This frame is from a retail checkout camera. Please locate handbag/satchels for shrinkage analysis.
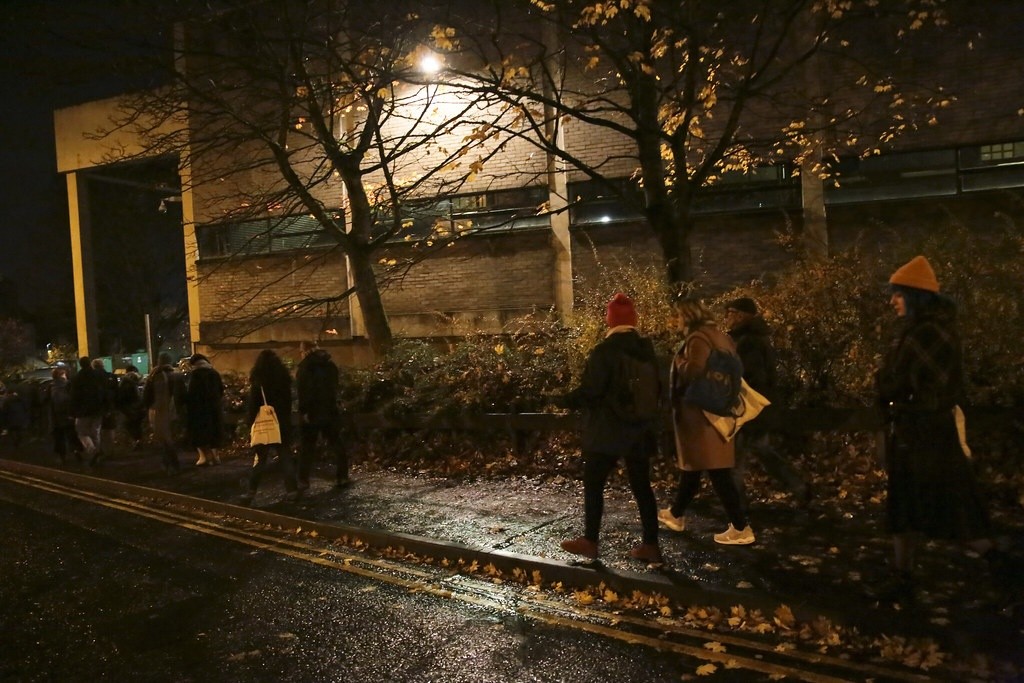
[702,376,771,442]
[909,404,971,473]
[251,405,281,447]
[740,344,785,391]
[143,369,157,404]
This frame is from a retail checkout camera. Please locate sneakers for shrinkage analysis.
[657,509,685,531]
[714,522,755,545]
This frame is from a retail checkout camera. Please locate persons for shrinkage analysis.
[296,341,348,486]
[657,296,756,545]
[560,294,663,563]
[725,298,821,510]
[46,357,145,465]
[0,376,44,447]
[143,353,225,466]
[863,256,991,601]
[240,350,297,500]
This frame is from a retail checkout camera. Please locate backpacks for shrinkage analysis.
[50,380,68,404]
[683,331,746,437]
[134,383,144,397]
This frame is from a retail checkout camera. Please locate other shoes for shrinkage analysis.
[861,569,914,597]
[960,585,999,611]
[59,450,82,466]
[624,541,657,561]
[196,456,222,465]
[296,474,309,488]
[337,477,351,489]
[561,536,603,562]
[132,438,144,452]
[88,449,105,468]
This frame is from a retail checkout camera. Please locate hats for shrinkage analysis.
[724,298,755,315]
[607,293,640,329]
[888,256,940,292]
